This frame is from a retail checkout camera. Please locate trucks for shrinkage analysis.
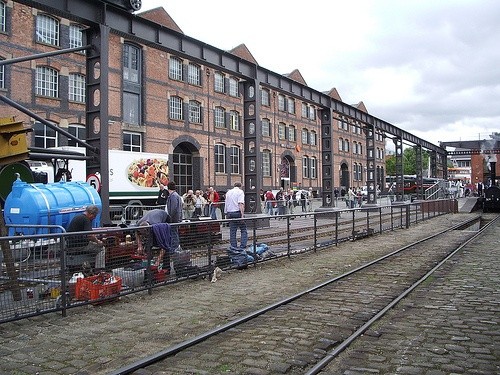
[27,146,170,221]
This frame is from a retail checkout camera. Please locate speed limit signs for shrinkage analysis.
[86,174,101,192]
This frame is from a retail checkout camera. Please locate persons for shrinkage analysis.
[260,187,313,219]
[65,205,103,254]
[135,209,173,275]
[224,182,247,248]
[158,184,169,211]
[341,187,361,207]
[181,187,219,220]
[334,187,339,198]
[458,179,485,198]
[165,181,182,252]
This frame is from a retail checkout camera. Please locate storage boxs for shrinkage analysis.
[112,267,146,287]
[75,272,121,306]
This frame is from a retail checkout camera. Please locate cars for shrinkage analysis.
[359,186,381,201]
